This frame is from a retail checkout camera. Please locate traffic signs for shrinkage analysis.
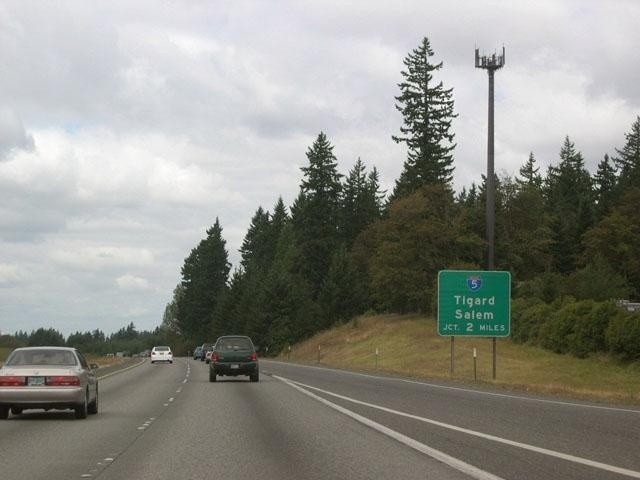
[438,269,512,338]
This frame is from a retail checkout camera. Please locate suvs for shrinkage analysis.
[209,335,259,382]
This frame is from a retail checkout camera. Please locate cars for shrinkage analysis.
[151,345,173,364]
[193,342,213,364]
[0,346,99,419]
